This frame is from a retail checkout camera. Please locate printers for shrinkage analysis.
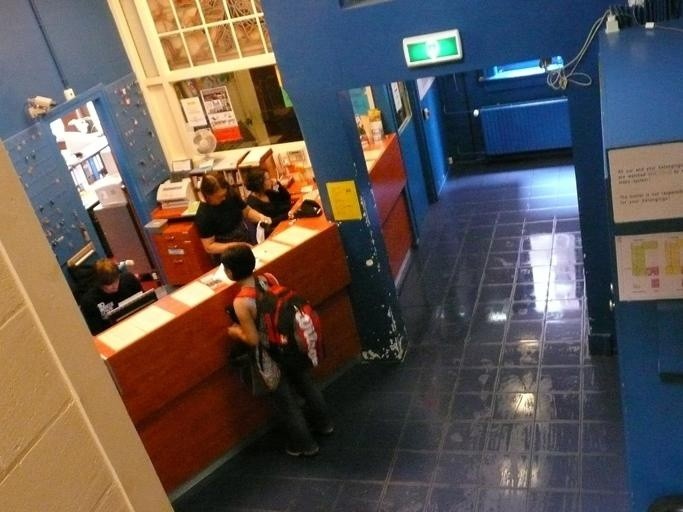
[156,159,197,210]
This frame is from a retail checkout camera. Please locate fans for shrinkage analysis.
[193,129,217,167]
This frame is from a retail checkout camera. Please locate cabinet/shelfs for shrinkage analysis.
[63,133,123,214]
[149,221,213,287]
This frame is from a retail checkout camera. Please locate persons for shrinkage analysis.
[237,168,304,245]
[193,170,274,266]
[220,241,337,460]
[76,256,142,321]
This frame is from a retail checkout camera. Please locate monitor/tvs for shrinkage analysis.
[107,288,158,326]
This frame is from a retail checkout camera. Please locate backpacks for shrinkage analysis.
[236,273,323,368]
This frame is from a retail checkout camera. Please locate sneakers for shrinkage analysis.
[285,428,333,456]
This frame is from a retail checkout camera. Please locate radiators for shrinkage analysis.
[478,96,573,157]
